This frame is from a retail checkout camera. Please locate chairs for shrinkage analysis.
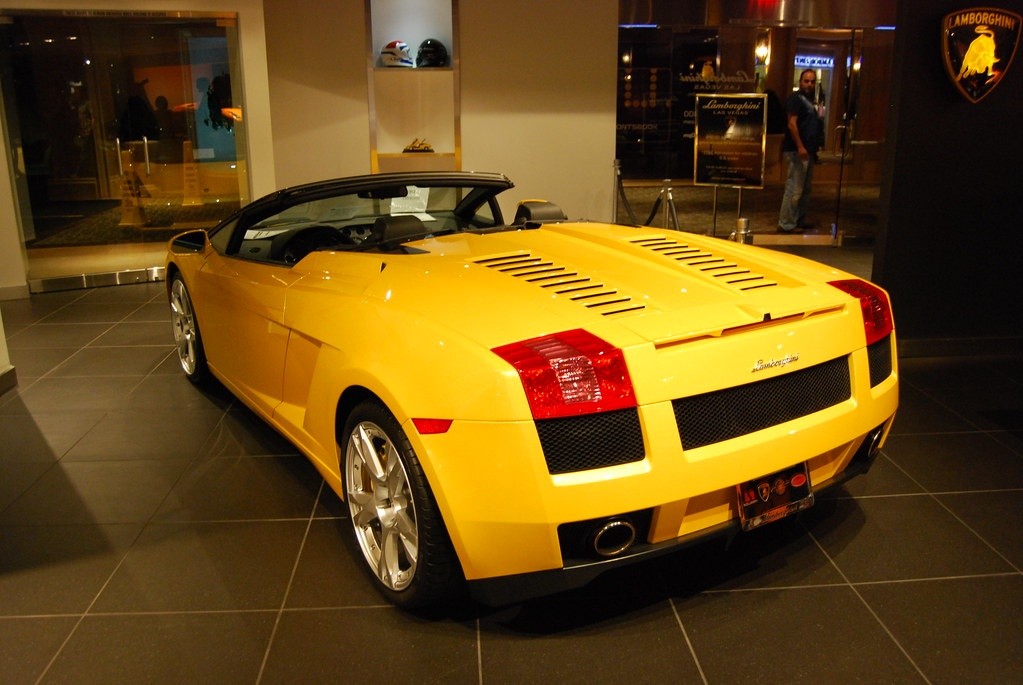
[372,213,423,245]
[514,199,565,225]
[273,224,358,259]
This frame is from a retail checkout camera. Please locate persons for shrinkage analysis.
[75,76,215,193]
[777,68,821,233]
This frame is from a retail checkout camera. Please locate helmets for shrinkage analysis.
[415,39,447,67]
[381,41,413,68]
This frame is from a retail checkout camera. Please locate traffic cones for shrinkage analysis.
[182,140,204,206]
[118,150,150,227]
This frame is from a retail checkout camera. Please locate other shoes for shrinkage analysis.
[776,217,813,235]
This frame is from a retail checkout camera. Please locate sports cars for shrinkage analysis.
[406,138,432,150]
[165,171,900,619]
